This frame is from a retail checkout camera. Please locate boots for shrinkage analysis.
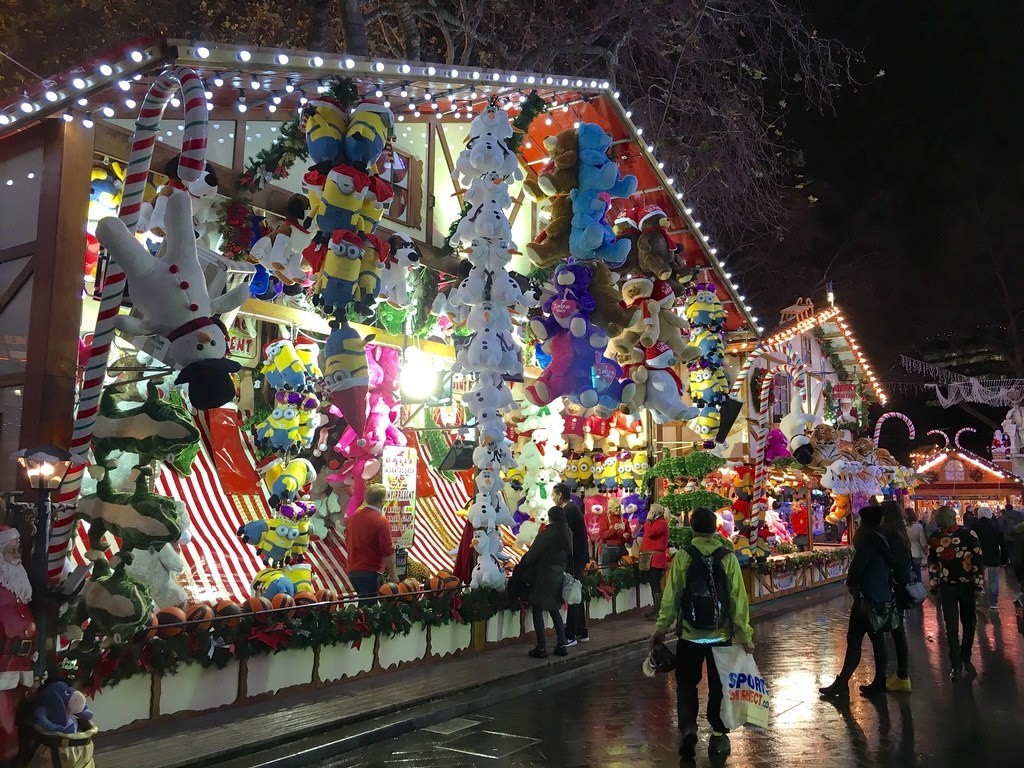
[648,593,663,621]
[645,593,660,618]
[860,677,886,692]
[819,675,849,694]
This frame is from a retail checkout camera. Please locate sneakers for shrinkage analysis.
[528,647,547,657]
[567,639,577,646]
[576,629,589,642]
[553,643,567,656]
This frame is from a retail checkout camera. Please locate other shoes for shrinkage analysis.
[708,734,730,756]
[950,668,961,681]
[989,605,999,609]
[886,674,911,693]
[679,729,698,757]
[965,662,976,674]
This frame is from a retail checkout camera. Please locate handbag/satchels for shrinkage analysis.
[639,551,662,571]
[868,598,909,632]
[527,587,551,609]
[563,572,582,604]
[712,646,768,730]
[904,581,927,604]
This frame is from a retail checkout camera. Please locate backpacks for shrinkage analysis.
[682,544,734,629]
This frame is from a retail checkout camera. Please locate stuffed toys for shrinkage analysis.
[63,71,921,639]
[25,681,96,733]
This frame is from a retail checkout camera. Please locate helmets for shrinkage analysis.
[966,506,973,513]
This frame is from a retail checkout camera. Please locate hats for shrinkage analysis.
[858,506,882,524]
[691,507,717,536]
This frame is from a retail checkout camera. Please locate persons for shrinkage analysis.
[652,507,755,758]
[551,483,590,647]
[345,483,400,608]
[821,500,1023,698]
[640,504,671,621]
[508,506,573,656]
[600,497,632,566]
[0,524,43,768]
[991,429,1011,460]
